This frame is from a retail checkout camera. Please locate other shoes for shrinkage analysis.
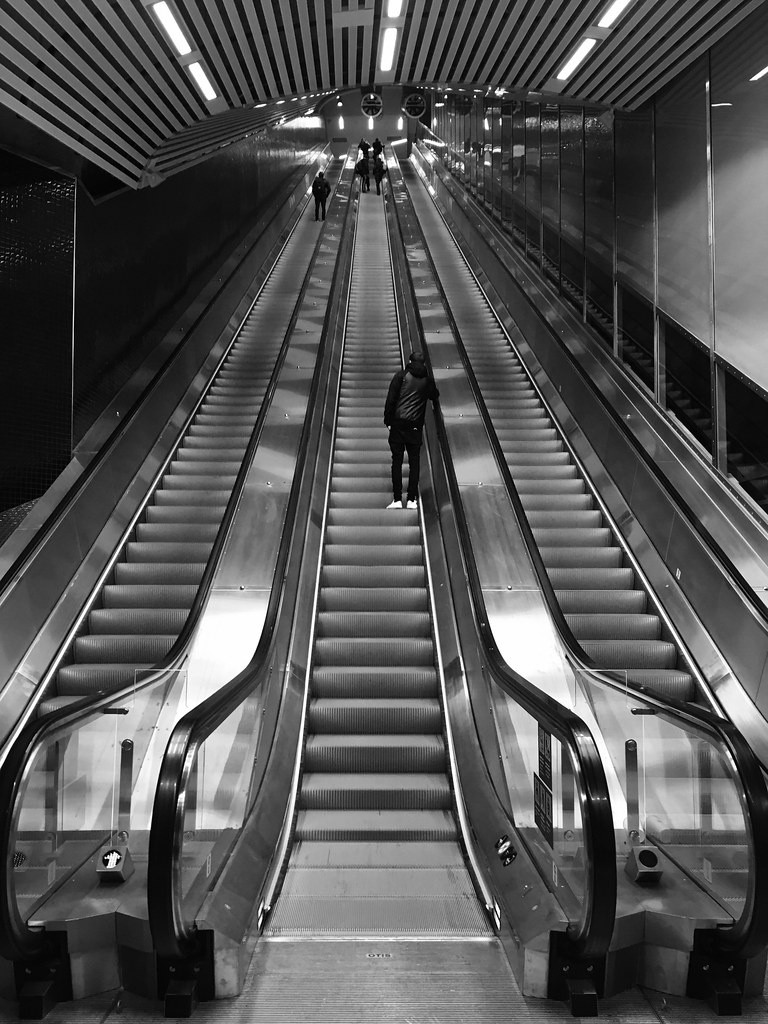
[362,189,372,193]
[406,499,418,509]
[315,220,327,222]
[385,500,403,509]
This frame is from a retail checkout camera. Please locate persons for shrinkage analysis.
[355,155,371,193]
[312,171,331,221]
[372,158,388,196]
[383,352,440,510]
[358,137,371,159]
[372,138,382,162]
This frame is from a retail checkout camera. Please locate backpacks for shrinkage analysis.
[355,161,363,174]
[315,184,326,199]
